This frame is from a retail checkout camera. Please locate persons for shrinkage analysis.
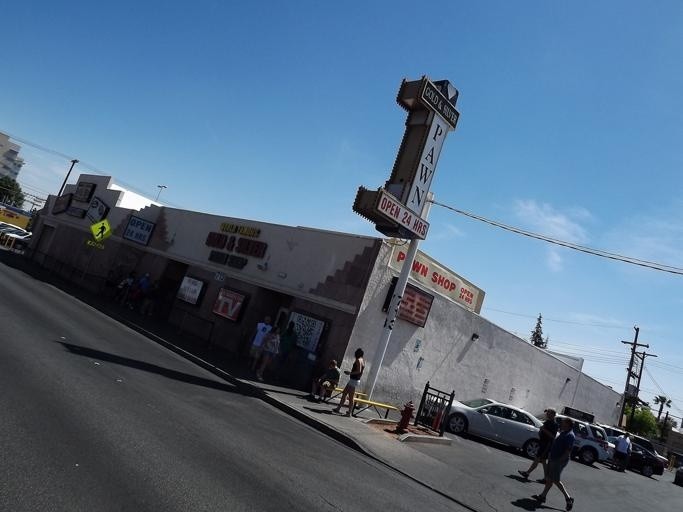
[247,312,298,382]
[307,360,340,404]
[332,348,364,417]
[115,271,161,317]
[611,432,633,472]
[531,418,574,511]
[518,407,559,484]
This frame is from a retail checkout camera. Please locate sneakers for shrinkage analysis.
[531,494,546,503]
[518,471,529,478]
[536,479,546,484]
[566,497,574,511]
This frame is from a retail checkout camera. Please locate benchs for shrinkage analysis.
[324,387,398,418]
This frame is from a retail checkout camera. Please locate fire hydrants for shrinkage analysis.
[396,401,416,434]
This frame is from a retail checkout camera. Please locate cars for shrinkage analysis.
[424,396,546,459]
[0,220,34,251]
[538,413,609,467]
[599,424,669,479]
